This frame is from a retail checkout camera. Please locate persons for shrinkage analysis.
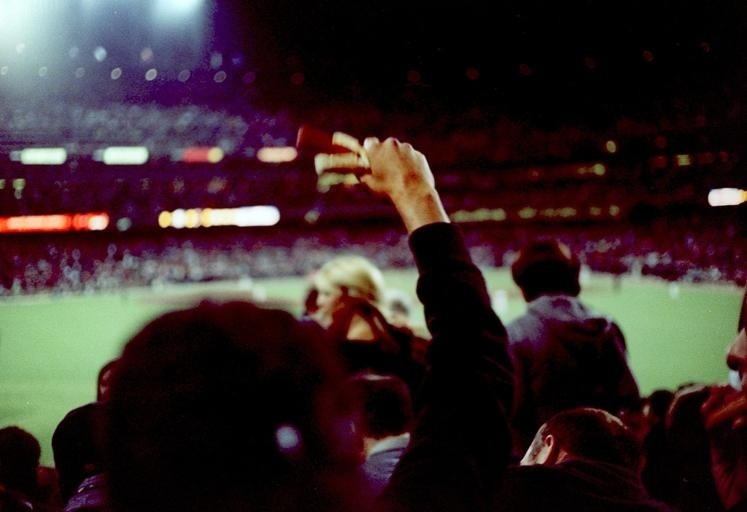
[1,136,747,511]
